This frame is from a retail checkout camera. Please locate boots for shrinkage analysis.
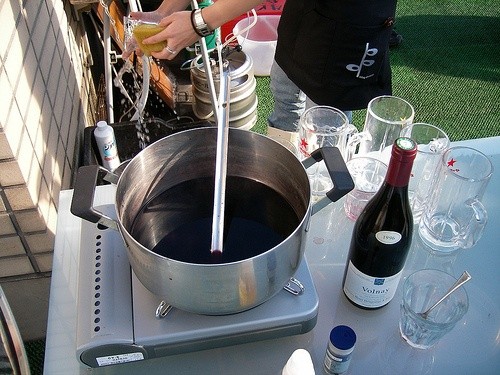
[267,126,299,158]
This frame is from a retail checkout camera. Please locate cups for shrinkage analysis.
[297,106,359,197]
[345,95,414,167]
[343,156,389,222]
[417,145,495,257]
[398,268,470,350]
[398,123,451,224]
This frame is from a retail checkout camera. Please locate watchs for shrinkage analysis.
[194,7,215,37]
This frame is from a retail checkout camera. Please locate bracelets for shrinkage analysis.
[190,9,206,37]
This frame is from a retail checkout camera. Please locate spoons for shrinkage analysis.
[404,271,471,336]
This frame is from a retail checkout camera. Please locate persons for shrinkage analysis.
[120,0,399,149]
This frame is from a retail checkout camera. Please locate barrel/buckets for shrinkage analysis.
[233,15,281,77]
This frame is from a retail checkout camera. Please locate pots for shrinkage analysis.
[69,127,355,316]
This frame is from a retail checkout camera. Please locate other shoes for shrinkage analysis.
[389,31,402,48]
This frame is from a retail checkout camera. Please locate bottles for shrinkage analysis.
[323,324,357,375]
[94,121,120,173]
[341,138,417,311]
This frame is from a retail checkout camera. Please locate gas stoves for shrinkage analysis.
[74,203,320,372]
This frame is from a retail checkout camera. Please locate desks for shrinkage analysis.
[42,135,500,375]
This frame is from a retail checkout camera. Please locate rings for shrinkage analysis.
[165,45,176,55]
